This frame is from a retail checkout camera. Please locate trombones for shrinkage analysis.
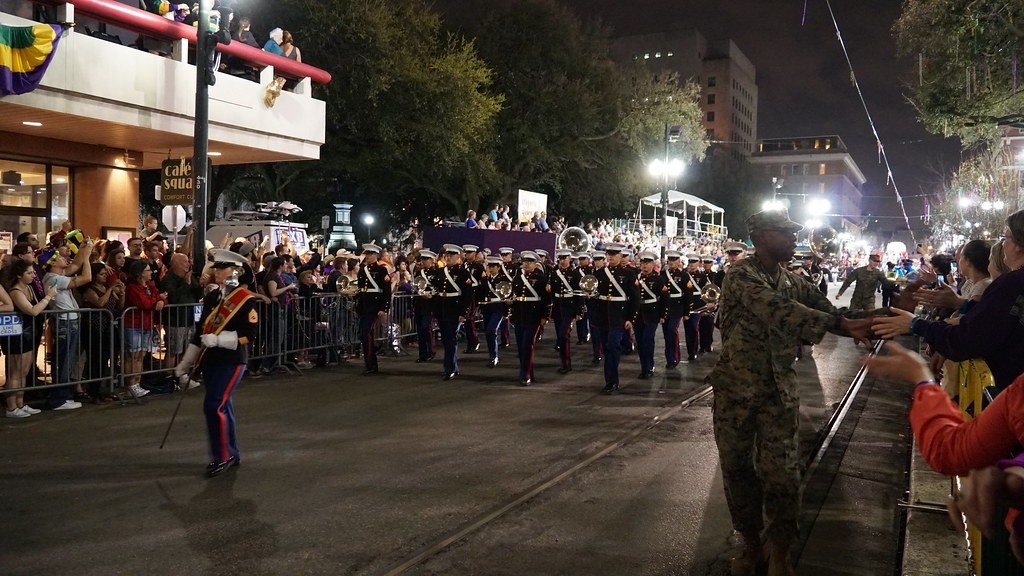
[701,283,722,304]
[414,277,427,294]
[497,281,513,298]
[579,275,599,293]
[336,275,352,293]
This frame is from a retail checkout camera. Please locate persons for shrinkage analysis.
[836,254,894,346]
[704,213,895,576]
[465,203,748,258]
[1,231,359,418]
[353,242,833,394]
[176,249,259,479]
[138,0,304,95]
[865,209,1024,575]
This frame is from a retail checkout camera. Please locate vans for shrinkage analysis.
[203,199,311,261]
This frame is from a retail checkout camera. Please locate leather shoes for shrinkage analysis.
[206,456,234,477]
[360,335,713,395]
[207,458,240,469]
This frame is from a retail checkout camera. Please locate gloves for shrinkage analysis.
[200,330,238,350]
[175,343,201,377]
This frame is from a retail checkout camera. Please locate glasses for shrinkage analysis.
[998,232,1017,242]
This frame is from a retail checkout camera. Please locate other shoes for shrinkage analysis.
[766,538,794,576]
[128,383,150,398]
[88,393,112,405]
[731,529,768,576]
[243,347,363,378]
[52,401,82,410]
[854,338,859,344]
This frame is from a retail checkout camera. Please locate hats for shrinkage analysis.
[870,255,881,262]
[363,241,749,266]
[902,259,913,265]
[39,249,59,268]
[748,206,803,233]
[210,249,249,268]
[153,235,168,241]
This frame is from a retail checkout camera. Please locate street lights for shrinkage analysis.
[365,215,376,243]
[771,176,785,206]
[650,124,684,267]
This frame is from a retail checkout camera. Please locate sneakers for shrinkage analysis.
[6,407,31,418]
[20,404,42,415]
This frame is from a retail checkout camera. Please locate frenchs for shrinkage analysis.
[559,226,590,259]
[808,223,841,289]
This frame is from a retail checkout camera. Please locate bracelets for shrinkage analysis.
[835,315,843,332]
[915,379,937,387]
[910,315,921,332]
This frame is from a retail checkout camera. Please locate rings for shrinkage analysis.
[930,300,933,302]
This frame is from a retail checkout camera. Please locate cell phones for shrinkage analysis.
[937,273,945,288]
[950,260,959,280]
[85,236,90,245]
